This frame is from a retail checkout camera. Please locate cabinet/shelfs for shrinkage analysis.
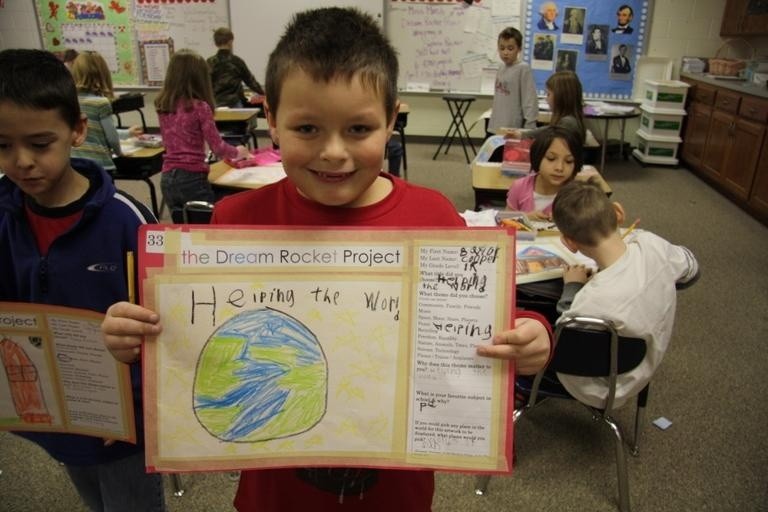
[680,75,768,216]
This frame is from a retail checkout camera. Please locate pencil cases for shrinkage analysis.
[495,212,538,241]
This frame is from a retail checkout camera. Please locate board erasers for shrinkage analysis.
[430,89,444,91]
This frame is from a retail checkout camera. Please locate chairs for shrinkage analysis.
[474,318,647,512]
[170,201,215,224]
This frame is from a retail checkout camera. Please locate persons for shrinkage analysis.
[505,127,627,227]
[505,69,587,166]
[0,49,166,510]
[515,181,698,415]
[535,2,634,76]
[206,28,264,146]
[63,50,78,83]
[153,49,254,224]
[484,26,537,160]
[70,51,143,184]
[101,6,557,511]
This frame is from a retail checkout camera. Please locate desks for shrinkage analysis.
[212,107,261,150]
[433,97,476,164]
[516,274,649,456]
[207,152,287,205]
[393,103,411,182]
[472,161,612,212]
[109,133,166,222]
[585,129,601,165]
[108,91,147,133]
[242,89,279,149]
[538,104,641,176]
[483,108,553,137]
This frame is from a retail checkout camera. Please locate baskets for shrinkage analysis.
[709,38,756,76]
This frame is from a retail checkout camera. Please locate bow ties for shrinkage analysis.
[616,27,625,30]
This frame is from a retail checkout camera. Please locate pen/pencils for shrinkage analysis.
[512,219,532,231]
[621,218,641,240]
[127,252,135,304]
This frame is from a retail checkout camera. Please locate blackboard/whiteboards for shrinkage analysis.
[383,0,526,99]
[32,0,233,93]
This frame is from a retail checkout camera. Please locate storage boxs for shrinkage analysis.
[633,131,683,168]
[640,106,687,137]
[642,79,691,109]
